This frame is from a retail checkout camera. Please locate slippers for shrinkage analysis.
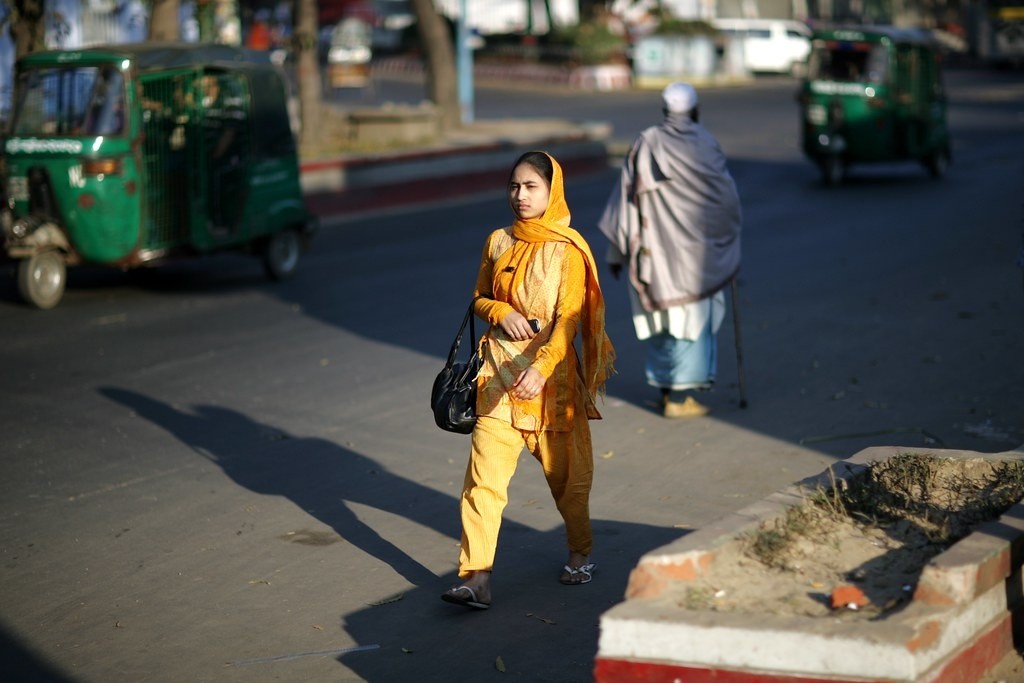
[560,561,597,588]
[440,585,491,612]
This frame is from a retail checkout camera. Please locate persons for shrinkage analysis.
[441,151,618,608]
[596,83,742,418]
[88,74,221,149]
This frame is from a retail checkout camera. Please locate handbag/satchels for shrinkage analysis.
[426,292,493,437]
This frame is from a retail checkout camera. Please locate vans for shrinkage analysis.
[712,15,814,75]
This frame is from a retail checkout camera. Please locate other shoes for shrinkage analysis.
[660,389,710,421]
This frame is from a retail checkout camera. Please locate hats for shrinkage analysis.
[662,82,698,114]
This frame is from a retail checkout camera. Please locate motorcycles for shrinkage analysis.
[787,23,953,192]
[0,43,313,314]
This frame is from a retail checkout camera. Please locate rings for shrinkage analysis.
[532,387,537,392]
